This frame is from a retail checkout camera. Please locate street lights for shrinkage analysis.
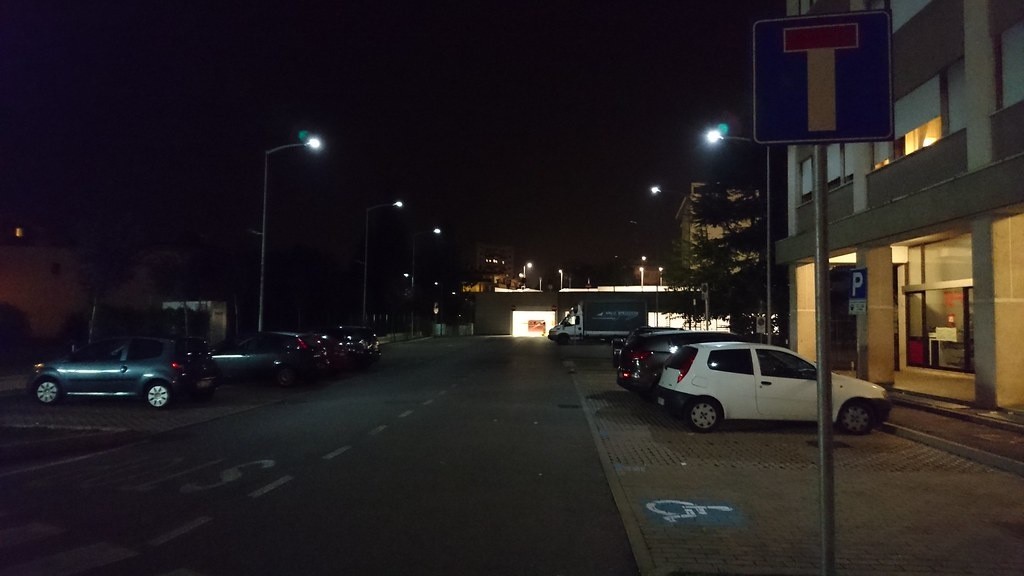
[639,266,645,291]
[408,228,443,337]
[558,269,563,290]
[359,200,402,325]
[706,130,773,347]
[659,266,664,286]
[255,134,322,331]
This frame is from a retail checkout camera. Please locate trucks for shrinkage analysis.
[548,299,648,344]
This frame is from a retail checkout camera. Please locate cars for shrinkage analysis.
[614,331,757,404]
[324,326,381,364]
[657,343,892,437]
[210,332,328,386]
[31,335,221,412]
